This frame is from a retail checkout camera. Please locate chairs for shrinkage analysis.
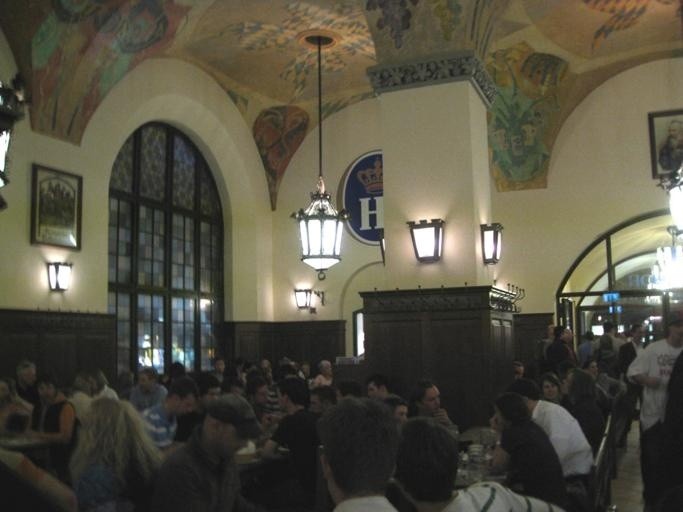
[571,413,615,511]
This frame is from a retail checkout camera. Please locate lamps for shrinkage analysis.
[481,223,503,263]
[295,284,325,314]
[644,164,683,304]
[290,175,350,280]
[406,219,443,264]
[47,263,74,291]
[0,86,27,209]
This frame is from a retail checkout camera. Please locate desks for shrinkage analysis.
[234,439,292,469]
[456,425,500,482]
[1,429,58,452]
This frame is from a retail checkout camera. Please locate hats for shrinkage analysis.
[208,394,261,434]
[669,311,683,327]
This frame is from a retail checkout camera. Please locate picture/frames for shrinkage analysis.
[29,162,84,252]
[647,108,683,178]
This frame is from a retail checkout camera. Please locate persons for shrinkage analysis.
[318,396,402,512]
[1,356,363,512]
[366,374,454,427]
[394,415,568,512]
[487,311,682,512]
[658,120,683,171]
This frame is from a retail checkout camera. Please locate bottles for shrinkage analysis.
[264,416,272,441]
